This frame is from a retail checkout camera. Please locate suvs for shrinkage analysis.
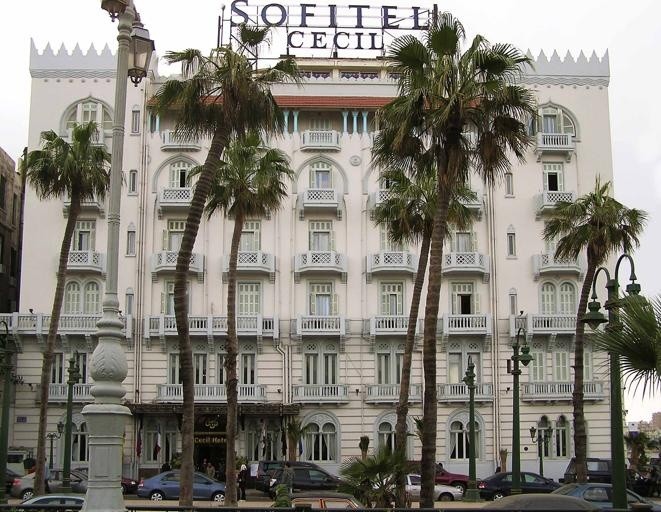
[395,461,471,496]
[563,457,634,483]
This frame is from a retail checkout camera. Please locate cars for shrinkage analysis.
[372,474,463,502]
[136,468,226,502]
[477,471,561,501]
[481,483,661,512]
[5,466,137,512]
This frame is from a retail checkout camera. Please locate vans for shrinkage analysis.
[255,460,367,510]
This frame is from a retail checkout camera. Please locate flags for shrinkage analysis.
[281,426,288,456]
[298,424,303,456]
[153,423,161,461]
[136,423,144,458]
[261,421,267,456]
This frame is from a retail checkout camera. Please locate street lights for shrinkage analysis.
[89,0,155,404]
[463,355,478,488]
[0,321,17,495]
[529,426,553,476]
[582,254,650,505]
[510,327,533,487]
[63,350,82,478]
[45,421,64,468]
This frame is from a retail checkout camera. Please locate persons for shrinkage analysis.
[647,464,661,498]
[237,463,248,501]
[44,456,51,493]
[206,462,216,479]
[281,462,296,494]
